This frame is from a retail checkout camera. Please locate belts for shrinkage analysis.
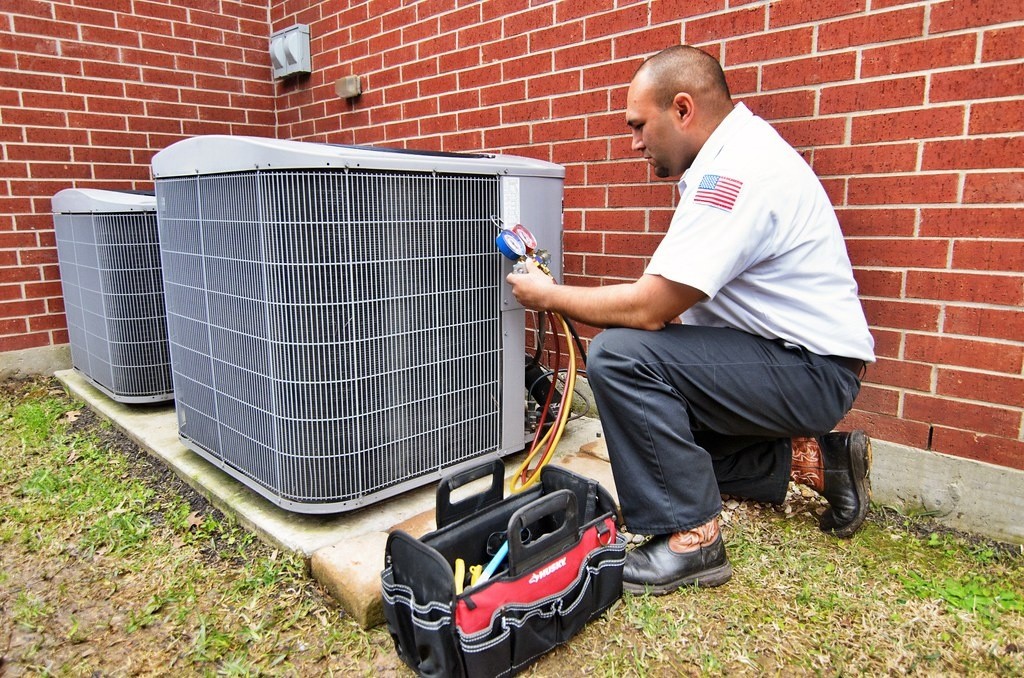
[828,354,867,383]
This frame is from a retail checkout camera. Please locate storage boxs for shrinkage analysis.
[379,458,627,678]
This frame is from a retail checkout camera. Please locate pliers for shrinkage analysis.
[454,558,483,599]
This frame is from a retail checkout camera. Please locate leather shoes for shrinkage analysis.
[625,535,730,594]
[817,432,872,537]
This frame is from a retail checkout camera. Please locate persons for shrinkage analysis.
[506,45,876,596]
